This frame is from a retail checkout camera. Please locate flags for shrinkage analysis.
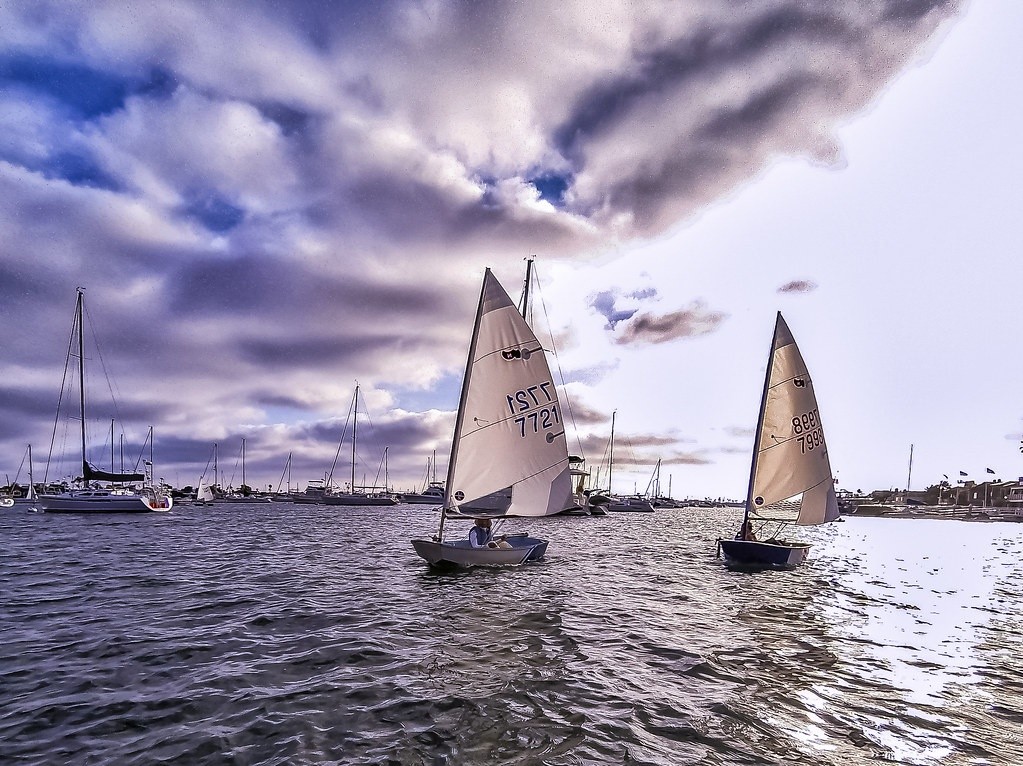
[960,471,968,476]
[987,468,995,474]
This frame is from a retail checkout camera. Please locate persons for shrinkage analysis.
[469,519,513,549]
[741,522,792,546]
[969,503,972,514]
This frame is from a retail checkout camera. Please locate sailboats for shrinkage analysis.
[32,284,176,515]
[408,264,581,574]
[714,308,840,574]
[0,252,747,518]
[319,380,400,506]
[906,443,928,505]
